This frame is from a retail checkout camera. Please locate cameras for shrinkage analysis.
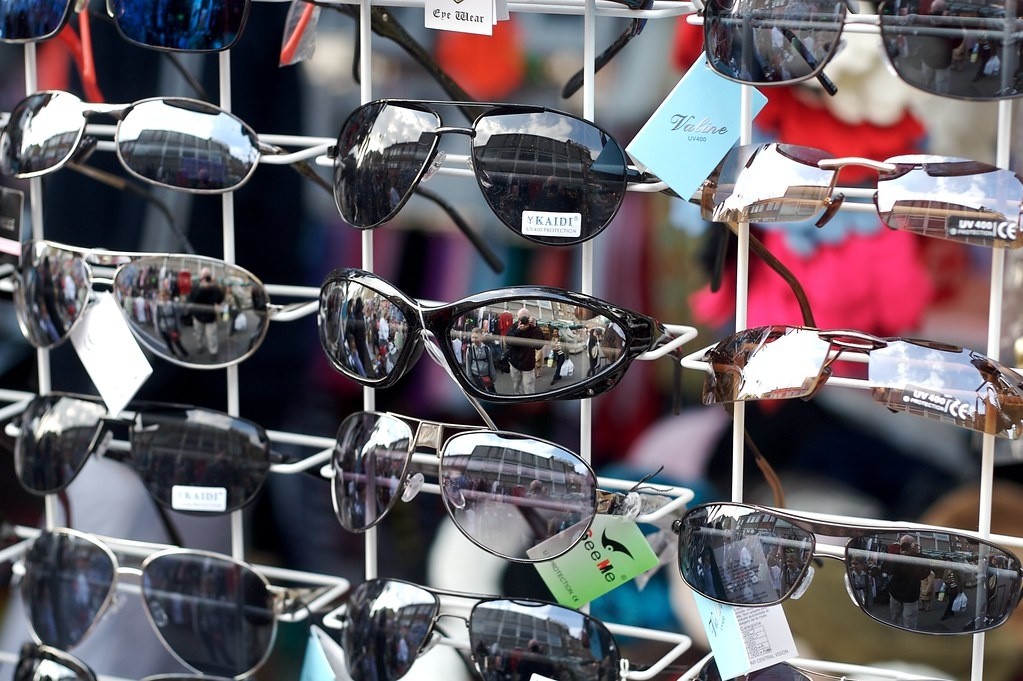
[205,275,211,282]
[932,11,943,16]
[521,316,528,325]
[900,542,911,551]
[549,184,558,193]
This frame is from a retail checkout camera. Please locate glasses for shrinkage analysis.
[342,577,690,680]
[671,504,1023,637]
[14,393,334,517]
[320,413,686,592]
[13,239,315,370]
[693,651,847,681]
[0,88,333,196]
[0,1,361,86]
[320,269,683,417]
[702,324,1022,508]
[13,642,232,681]
[701,144,1023,327]
[699,0,1023,103]
[326,99,819,330]
[20,526,335,679]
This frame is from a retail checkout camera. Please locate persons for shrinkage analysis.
[138,443,260,511]
[467,306,626,394]
[356,605,420,681]
[498,175,616,243]
[679,522,810,606]
[884,0,1023,96]
[117,264,266,360]
[850,535,1021,630]
[18,245,88,346]
[48,533,91,648]
[475,637,616,681]
[443,470,583,555]
[707,11,831,84]
[325,287,405,380]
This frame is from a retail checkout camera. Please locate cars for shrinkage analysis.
[559,328,587,354]
[551,657,596,680]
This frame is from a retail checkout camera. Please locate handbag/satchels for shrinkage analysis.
[480,376,492,388]
[180,314,192,327]
[169,332,178,341]
[500,357,510,375]
